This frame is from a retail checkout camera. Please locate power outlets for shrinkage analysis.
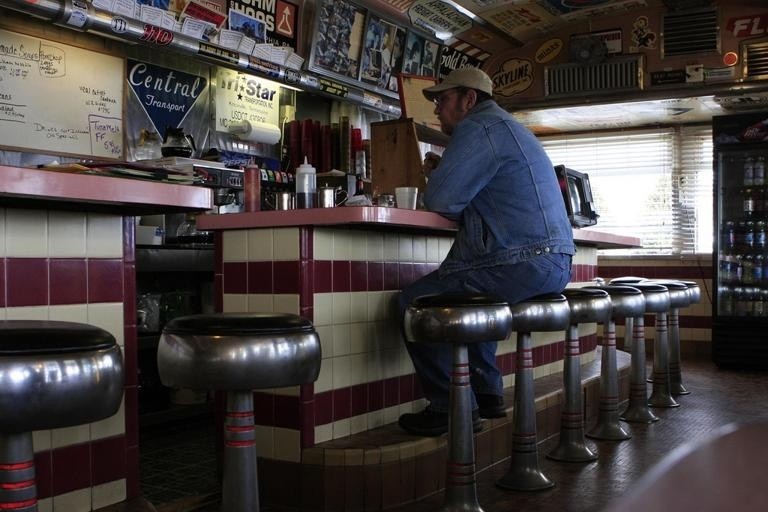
[685,65,704,82]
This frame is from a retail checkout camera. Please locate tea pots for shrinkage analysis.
[162,127,197,158]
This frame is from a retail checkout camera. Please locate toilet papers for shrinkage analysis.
[236,119,282,146]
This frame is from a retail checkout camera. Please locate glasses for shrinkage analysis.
[432,88,461,106]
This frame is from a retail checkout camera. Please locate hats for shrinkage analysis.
[423,67,494,102]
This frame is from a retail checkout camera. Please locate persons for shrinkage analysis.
[424,42,435,67]
[383,32,393,71]
[388,28,406,91]
[366,23,381,49]
[399,65,573,436]
[405,41,419,73]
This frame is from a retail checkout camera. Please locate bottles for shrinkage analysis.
[356,175,364,195]
[295,155,317,208]
[243,156,262,211]
[156,225,164,237]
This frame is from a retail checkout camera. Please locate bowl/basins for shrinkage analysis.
[213,192,234,206]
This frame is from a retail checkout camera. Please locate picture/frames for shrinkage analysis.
[227,8,267,44]
[177,1,228,35]
[398,26,444,80]
[308,1,370,90]
[568,26,624,61]
[396,72,441,138]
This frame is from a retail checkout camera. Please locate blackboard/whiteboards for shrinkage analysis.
[0,4,127,163]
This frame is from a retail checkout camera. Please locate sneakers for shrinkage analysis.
[399,405,482,436]
[473,392,507,420]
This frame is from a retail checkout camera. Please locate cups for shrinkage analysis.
[318,182,348,208]
[395,187,418,210]
[377,194,395,207]
[277,193,294,210]
[284,117,372,178]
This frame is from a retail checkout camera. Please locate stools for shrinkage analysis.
[1,319,126,510]
[494,290,571,491]
[544,275,702,462]
[155,313,321,509]
[400,291,513,510]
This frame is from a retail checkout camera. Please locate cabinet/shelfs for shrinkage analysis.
[370,116,453,211]
